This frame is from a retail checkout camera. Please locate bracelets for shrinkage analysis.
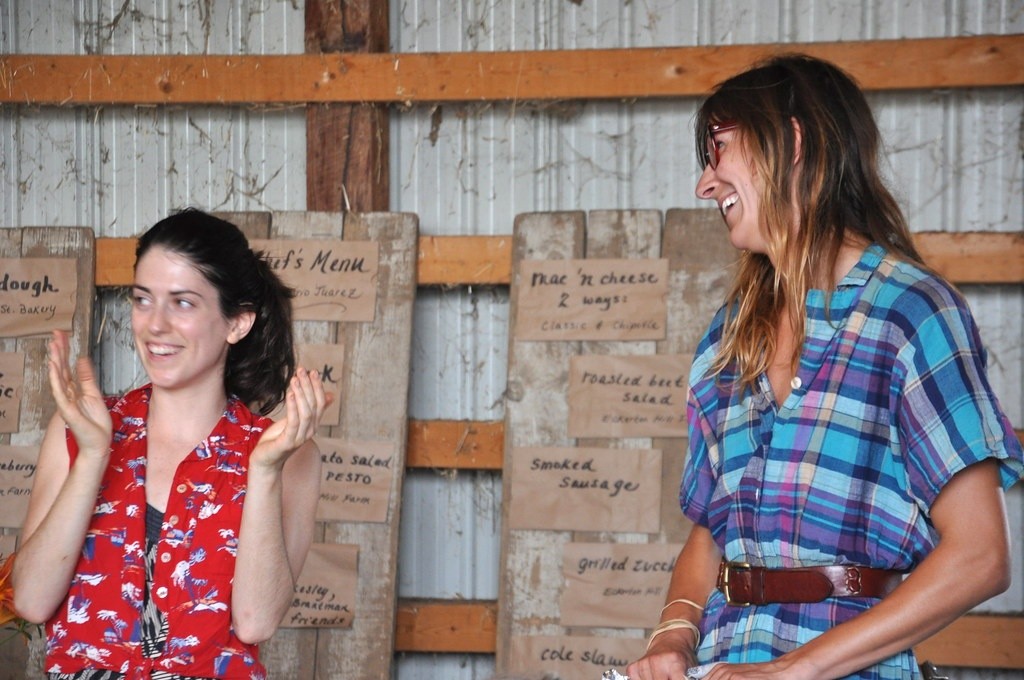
[661,599,704,615]
[647,619,700,649]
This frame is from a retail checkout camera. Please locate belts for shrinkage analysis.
[716,559,904,608]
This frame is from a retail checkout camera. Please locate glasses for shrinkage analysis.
[704,116,742,172]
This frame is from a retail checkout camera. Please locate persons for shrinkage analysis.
[626,52,1024,680]
[13,206,334,680]
